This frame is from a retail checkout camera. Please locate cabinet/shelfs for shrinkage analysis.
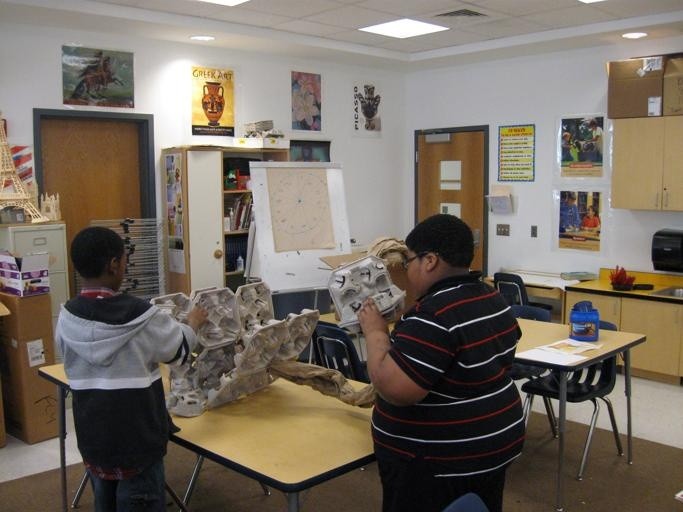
[564,290,621,375]
[620,296,683,387]
[160,146,294,297]
[0,222,72,364]
[609,116,682,214]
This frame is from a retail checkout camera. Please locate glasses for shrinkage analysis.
[401,249,436,272]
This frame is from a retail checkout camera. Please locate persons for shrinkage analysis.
[582,205,601,231]
[55,226,209,512]
[358,215,525,512]
[560,193,582,228]
[562,132,571,160]
[585,120,602,157]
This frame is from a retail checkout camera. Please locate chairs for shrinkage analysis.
[517,318,625,482]
[492,272,553,322]
[445,490,488,511]
[312,321,370,385]
[507,304,559,438]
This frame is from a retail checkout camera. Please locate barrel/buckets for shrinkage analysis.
[569,301,600,342]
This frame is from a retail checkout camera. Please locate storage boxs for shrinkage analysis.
[0,288,65,445]
[231,139,290,150]
[1,249,50,299]
[662,51,682,117]
[605,53,664,121]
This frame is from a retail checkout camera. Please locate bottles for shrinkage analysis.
[237,256,244,271]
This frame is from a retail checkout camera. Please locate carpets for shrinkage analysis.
[0,410,683,511]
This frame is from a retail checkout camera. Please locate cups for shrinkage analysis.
[568,308,599,341]
[223,216,230,232]
[200,81,225,125]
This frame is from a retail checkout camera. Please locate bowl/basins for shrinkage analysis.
[610,282,633,291]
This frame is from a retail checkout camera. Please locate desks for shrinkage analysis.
[484,270,592,324]
[310,309,648,510]
[0,302,12,449]
[37,350,378,511]
